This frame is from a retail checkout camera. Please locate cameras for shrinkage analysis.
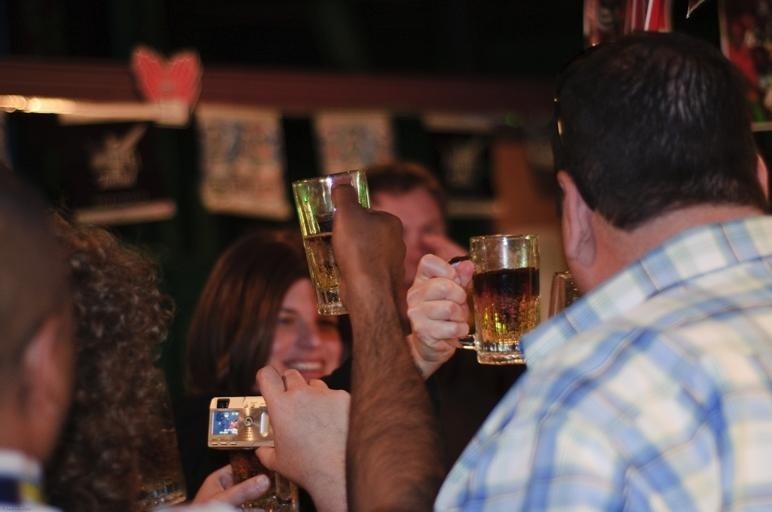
[208,396,275,449]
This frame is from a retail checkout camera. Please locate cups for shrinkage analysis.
[292,168,372,316]
[447,232,544,367]
[547,271,579,319]
[231,445,300,512]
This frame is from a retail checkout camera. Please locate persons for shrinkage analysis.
[1,175,349,511]
[330,143,606,511]
[176,227,349,419]
[41,220,179,511]
[352,161,545,467]
